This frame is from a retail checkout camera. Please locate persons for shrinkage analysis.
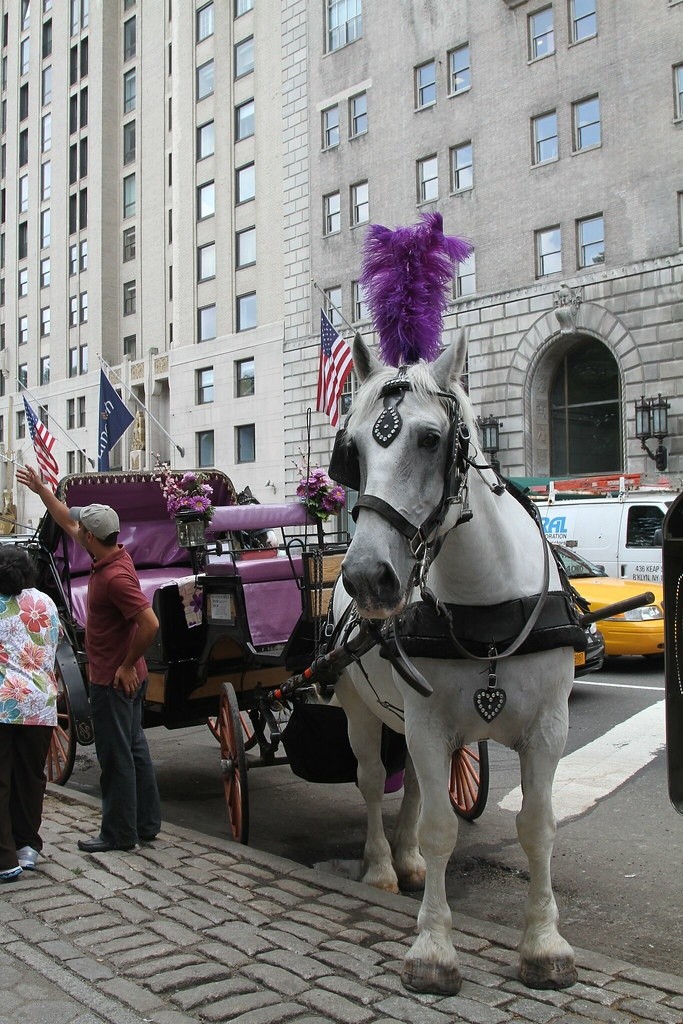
[15,464,161,853]
[0,543,65,879]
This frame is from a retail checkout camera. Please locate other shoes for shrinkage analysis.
[16,846,38,867]
[0,865,23,878]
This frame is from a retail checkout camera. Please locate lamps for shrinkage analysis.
[475,413,503,474]
[633,392,671,474]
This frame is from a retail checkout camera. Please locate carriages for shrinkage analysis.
[0,212,654,997]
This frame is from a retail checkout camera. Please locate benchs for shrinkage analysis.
[197,500,317,584]
[49,524,201,629]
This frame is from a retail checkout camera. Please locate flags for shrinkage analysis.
[97,368,136,472]
[316,308,356,427]
[23,394,60,494]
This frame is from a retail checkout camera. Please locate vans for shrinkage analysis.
[527,473,681,584]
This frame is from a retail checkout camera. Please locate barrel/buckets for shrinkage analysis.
[385,772,404,793]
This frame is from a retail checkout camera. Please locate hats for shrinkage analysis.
[68,503,120,540]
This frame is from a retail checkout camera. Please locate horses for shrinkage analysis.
[323,325,588,996]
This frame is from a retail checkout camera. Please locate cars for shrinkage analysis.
[551,542,665,678]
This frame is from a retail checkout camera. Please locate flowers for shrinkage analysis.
[149,449,215,525]
[288,445,347,524]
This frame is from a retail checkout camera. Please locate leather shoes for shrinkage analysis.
[139,834,157,838]
[78,837,135,851]
